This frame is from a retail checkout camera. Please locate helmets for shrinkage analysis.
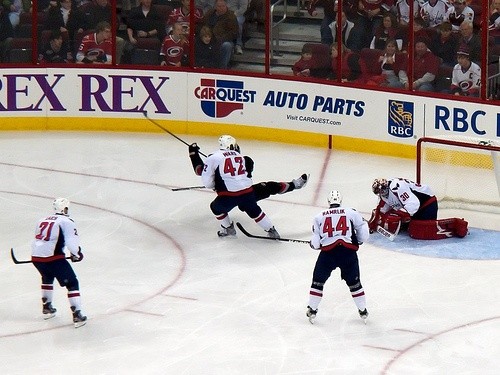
[218,135,237,150]
[372,179,389,196]
[53,198,68,214]
[327,190,343,204]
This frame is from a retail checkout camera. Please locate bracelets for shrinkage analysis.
[146,32,150,38]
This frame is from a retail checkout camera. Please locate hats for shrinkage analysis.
[456,43,470,56]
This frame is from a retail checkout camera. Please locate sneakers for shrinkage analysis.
[306,305,318,325]
[71,306,87,327]
[42,297,57,319]
[265,225,279,239]
[293,173,310,189]
[217,221,236,237]
[358,307,368,324]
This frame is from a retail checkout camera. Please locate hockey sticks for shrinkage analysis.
[350,207,401,242]
[11,248,70,264]
[142,110,208,158]
[155,182,206,191]
[236,222,311,245]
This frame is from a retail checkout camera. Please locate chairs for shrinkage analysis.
[10,4,174,64]
[303,0,500,95]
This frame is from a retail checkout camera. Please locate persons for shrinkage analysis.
[306,190,370,318]
[201,134,281,239]
[188,141,308,203]
[292,0,500,99]
[368,178,468,240]
[0,0,249,69]
[442,44,481,99]
[31,197,87,323]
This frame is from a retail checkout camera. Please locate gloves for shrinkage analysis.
[71,246,83,262]
[189,142,200,155]
